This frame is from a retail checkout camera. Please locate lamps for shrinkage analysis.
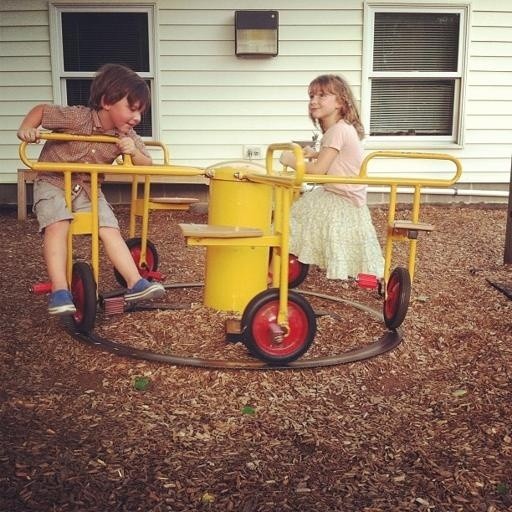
[234,10,279,55]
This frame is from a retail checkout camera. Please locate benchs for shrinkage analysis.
[17,167,211,223]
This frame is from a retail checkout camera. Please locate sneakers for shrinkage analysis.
[48,289,77,316]
[124,278,165,301]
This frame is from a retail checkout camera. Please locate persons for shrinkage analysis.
[265,75,386,288]
[18,59,167,317]
